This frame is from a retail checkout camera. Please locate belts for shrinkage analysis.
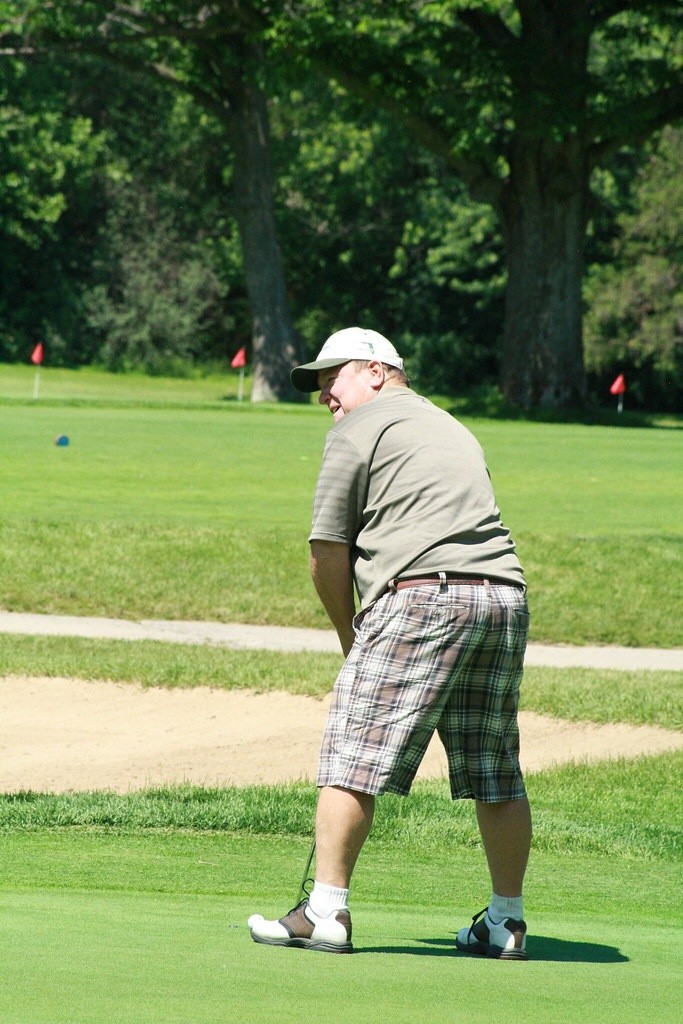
[396,577,516,585]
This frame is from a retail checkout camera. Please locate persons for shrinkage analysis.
[245,324,536,960]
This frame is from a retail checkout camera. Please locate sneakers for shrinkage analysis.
[455,913,533,960]
[248,902,354,953]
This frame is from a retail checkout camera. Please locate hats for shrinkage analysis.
[286,327,403,392]
